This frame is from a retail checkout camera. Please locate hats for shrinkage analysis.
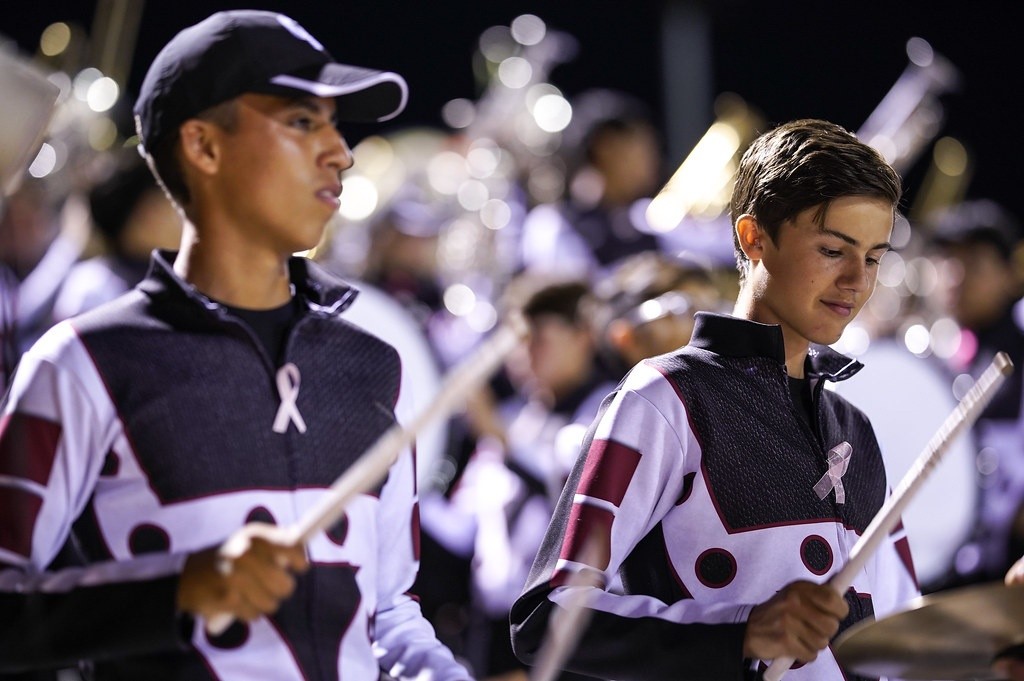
[134,10,408,151]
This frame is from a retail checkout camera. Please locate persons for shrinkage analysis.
[509,117,923,681]
[0,88,1024,681]
[0,8,478,681]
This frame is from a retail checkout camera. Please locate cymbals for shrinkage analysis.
[830,581,1024,681]
